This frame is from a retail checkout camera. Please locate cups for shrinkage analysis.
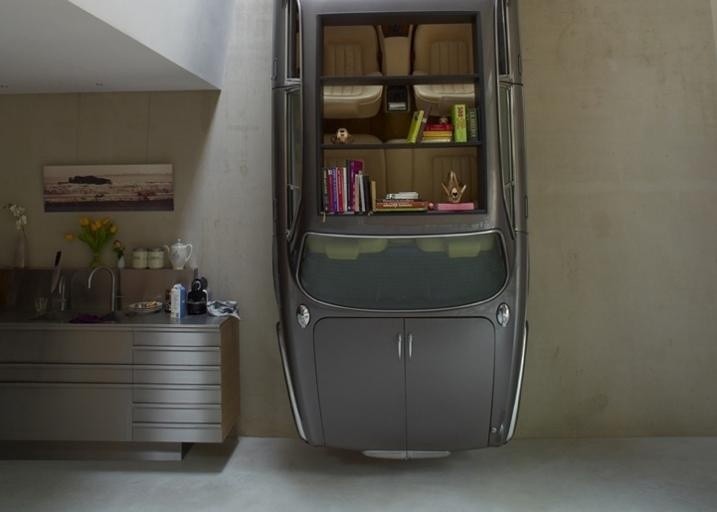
[34,297,49,313]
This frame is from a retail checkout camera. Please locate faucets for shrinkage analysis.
[86,265,125,313]
[58,275,68,310]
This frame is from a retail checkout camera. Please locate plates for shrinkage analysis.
[127,300,163,316]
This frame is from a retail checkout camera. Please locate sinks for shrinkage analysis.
[21,311,77,325]
[74,312,131,322]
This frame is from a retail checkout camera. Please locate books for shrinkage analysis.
[405,104,477,143]
[321,159,429,215]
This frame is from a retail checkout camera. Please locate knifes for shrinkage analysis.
[50,251,61,296]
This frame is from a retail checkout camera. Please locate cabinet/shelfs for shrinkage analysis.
[269,0,532,462]
[1,307,239,462]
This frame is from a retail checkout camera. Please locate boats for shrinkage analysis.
[69,176,112,185]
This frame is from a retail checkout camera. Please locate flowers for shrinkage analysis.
[64,213,127,269]
[5,202,31,233]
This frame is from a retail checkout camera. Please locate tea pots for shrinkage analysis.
[163,237,193,271]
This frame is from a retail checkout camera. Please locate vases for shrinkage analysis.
[15,233,29,270]
[87,255,108,275]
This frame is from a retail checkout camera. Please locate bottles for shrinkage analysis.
[133,247,149,269]
[148,247,165,269]
